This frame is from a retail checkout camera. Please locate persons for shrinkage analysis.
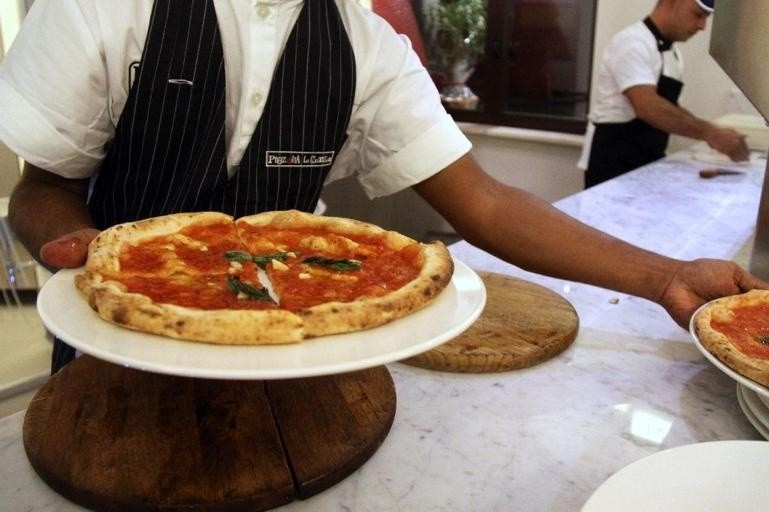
[577,1,750,189]
[1,1,768,378]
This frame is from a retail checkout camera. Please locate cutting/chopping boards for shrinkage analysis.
[23,353,396,512]
[395,270,579,375]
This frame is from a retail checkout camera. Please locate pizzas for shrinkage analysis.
[74,208,454,347]
[693,287,765,387]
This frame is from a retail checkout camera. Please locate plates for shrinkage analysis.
[735,380,769,441]
[694,153,755,166]
[581,439,769,511]
[690,293,769,398]
[37,253,486,380]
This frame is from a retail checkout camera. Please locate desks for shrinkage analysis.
[0,142,769,512]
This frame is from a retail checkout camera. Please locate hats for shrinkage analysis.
[698,0,715,12]
